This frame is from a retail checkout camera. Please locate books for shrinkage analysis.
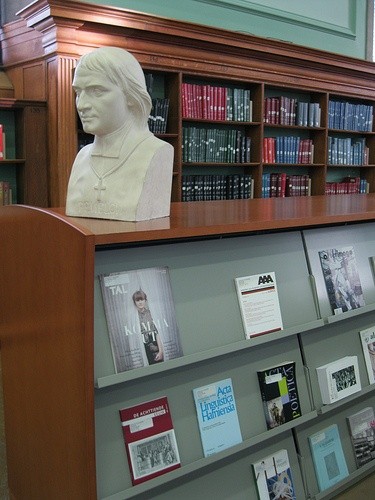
[318,245,366,315]
[119,396,181,487]
[182,128,250,163]
[235,272,283,340]
[250,449,297,500]
[250,101,252,121]
[257,361,302,431]
[148,98,169,133]
[0,181,12,205]
[193,378,243,458]
[262,136,314,164]
[99,266,183,373]
[0,124,6,160]
[262,172,311,198]
[346,407,375,469]
[181,84,250,121]
[308,424,349,493]
[182,175,254,202]
[327,137,369,165]
[327,177,369,194]
[329,101,373,131]
[359,326,375,384]
[264,96,321,127]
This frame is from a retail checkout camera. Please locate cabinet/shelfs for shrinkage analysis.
[0,0,375,500]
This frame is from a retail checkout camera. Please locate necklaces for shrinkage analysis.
[89,134,152,201]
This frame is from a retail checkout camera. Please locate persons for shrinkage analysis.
[66,47,174,222]
[271,403,280,423]
[132,290,164,365]
[332,370,356,392]
